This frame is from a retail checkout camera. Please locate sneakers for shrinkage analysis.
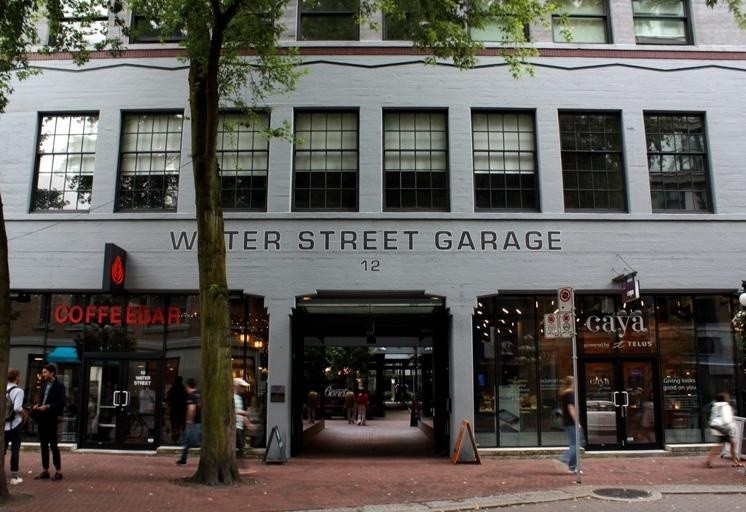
[732,464,743,467]
[51,472,62,481]
[35,470,49,480]
[9,477,22,486]
[567,469,582,475]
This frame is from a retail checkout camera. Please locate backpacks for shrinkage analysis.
[4,384,21,431]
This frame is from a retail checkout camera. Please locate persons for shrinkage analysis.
[308,390,319,423]
[344,387,357,424]
[34,364,66,482]
[174,379,205,465]
[701,391,744,467]
[234,377,256,459]
[168,376,187,445]
[356,389,368,425]
[395,381,400,402]
[559,375,587,474]
[4,369,30,485]
[637,394,654,443]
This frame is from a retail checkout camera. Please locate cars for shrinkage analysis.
[550,400,617,431]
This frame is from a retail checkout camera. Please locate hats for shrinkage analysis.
[232,378,249,388]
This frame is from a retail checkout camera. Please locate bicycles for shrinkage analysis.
[128,413,155,438]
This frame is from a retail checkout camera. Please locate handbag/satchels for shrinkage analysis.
[709,407,730,434]
